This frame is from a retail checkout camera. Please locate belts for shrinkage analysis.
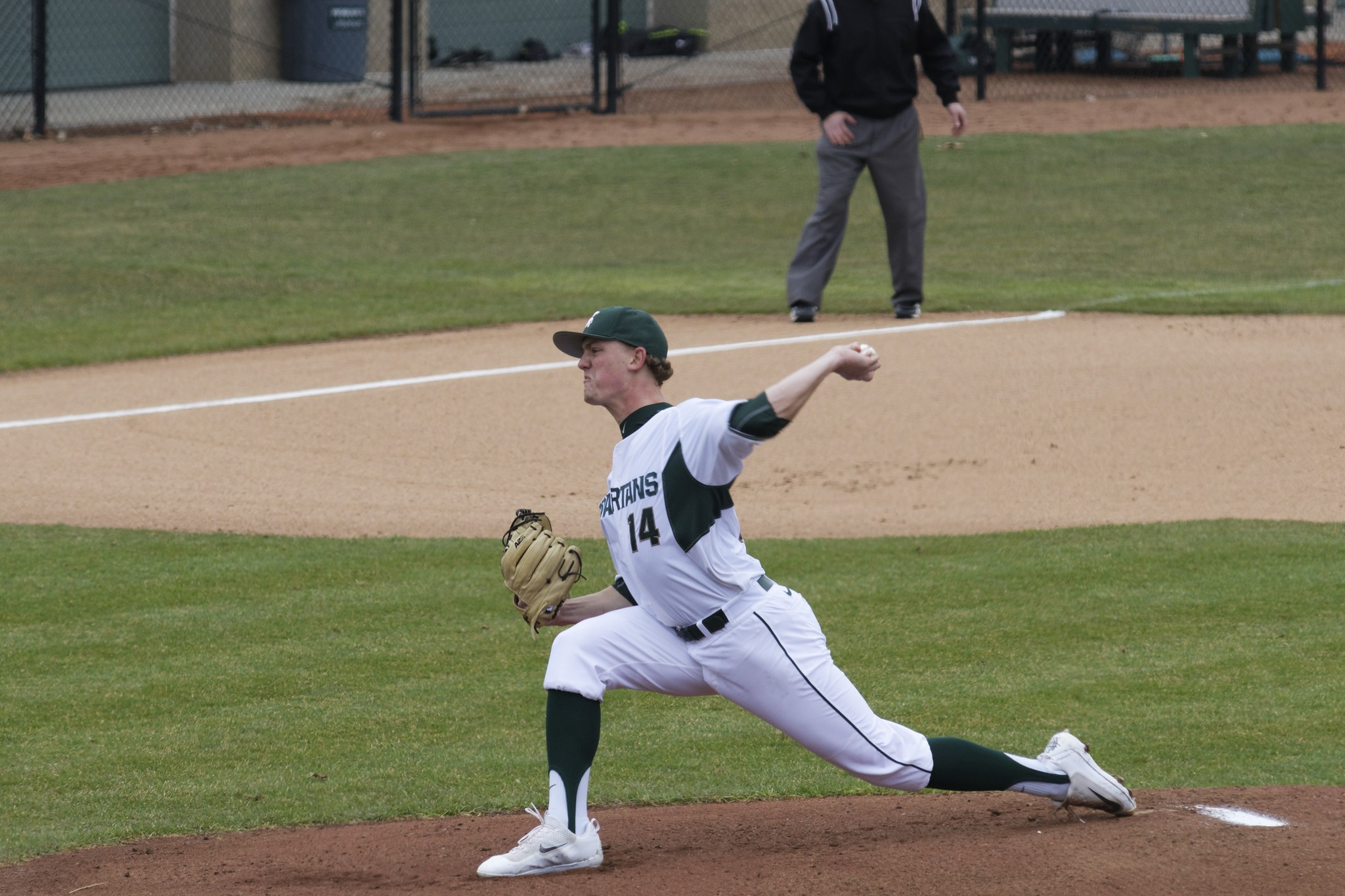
[669,572,774,642]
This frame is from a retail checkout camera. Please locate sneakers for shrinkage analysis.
[476,802,605,879]
[789,302,818,323]
[895,302,922,318]
[1037,729,1138,822]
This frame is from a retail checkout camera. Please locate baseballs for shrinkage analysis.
[860,343,877,359]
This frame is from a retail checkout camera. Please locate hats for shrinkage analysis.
[552,305,669,360]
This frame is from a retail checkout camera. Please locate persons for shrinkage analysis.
[476,306,1136,876]
[788,0,968,322]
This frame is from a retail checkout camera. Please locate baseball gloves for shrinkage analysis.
[498,508,583,629]
